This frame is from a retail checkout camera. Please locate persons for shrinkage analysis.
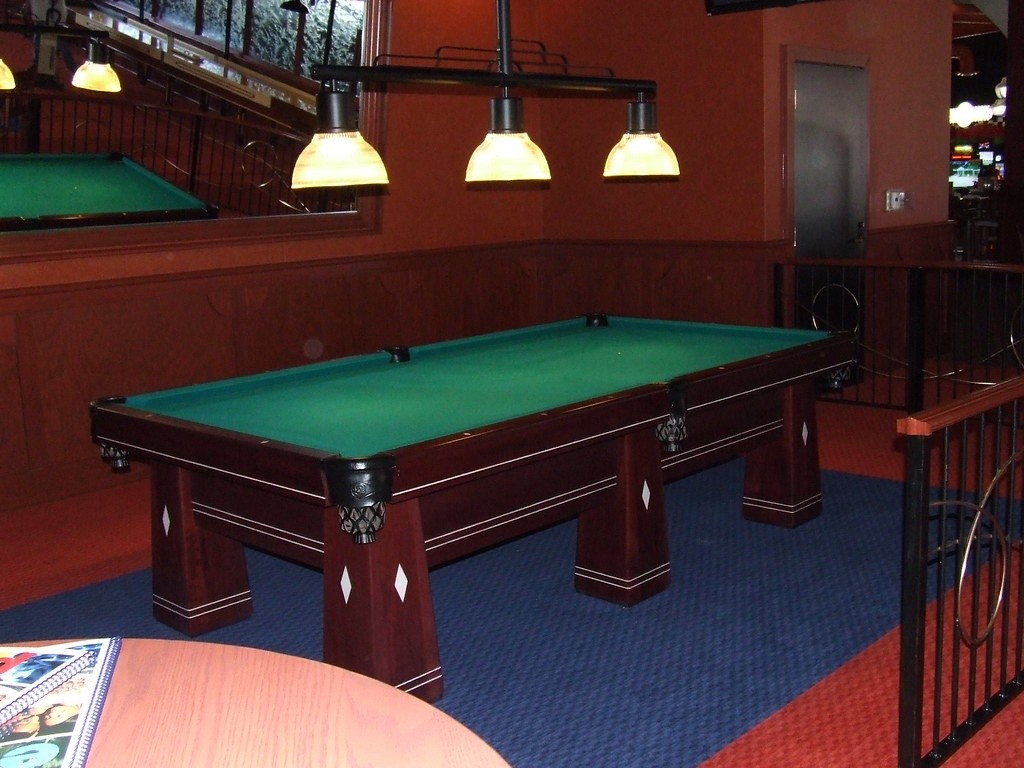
[8,686,89,739]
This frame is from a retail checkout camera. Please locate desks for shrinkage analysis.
[0,153,221,231]
[89,311,862,704]
[0,639,511,768]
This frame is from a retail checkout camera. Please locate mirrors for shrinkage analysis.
[0,0,395,266]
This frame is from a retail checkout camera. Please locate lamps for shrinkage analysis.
[462,87,552,181]
[602,92,681,179]
[71,38,122,92]
[291,79,391,189]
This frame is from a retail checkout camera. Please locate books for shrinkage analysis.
[0,636,97,724]
[0,637,123,768]
